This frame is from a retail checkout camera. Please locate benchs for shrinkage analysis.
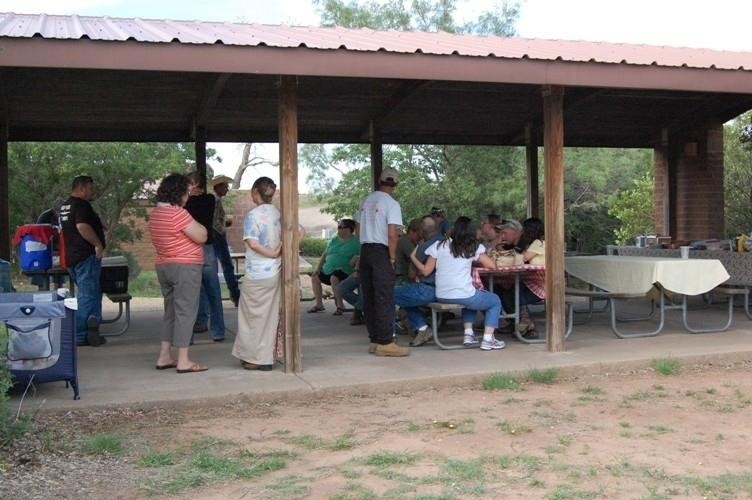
[98,291,135,343]
[428,302,480,350]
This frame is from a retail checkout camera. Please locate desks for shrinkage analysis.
[230,252,245,284]
[20,266,77,296]
[472,266,546,344]
[563,245,751,339]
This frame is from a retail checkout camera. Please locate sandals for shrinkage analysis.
[333,305,346,316]
[308,306,325,313]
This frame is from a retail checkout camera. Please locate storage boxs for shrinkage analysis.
[101,264,130,294]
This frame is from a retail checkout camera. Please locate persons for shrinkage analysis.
[33,199,64,289]
[60,176,106,347]
[209,172,240,310]
[233,177,282,370]
[149,173,211,371]
[184,170,224,340]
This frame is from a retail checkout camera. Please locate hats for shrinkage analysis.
[495,219,523,234]
[377,167,400,187]
[209,174,234,187]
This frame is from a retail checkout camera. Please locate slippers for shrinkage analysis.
[156,359,178,370]
[176,362,209,374]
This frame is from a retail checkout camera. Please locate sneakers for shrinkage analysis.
[409,327,434,347]
[368,335,409,357]
[472,311,539,339]
[194,322,225,341]
[351,315,363,325]
[463,333,479,346]
[240,359,273,371]
[76,315,106,347]
[479,338,506,351]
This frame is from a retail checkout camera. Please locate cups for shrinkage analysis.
[606,245,614,255]
[729,241,736,252]
[680,246,690,259]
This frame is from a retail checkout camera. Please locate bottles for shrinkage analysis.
[639,234,647,248]
[735,234,745,252]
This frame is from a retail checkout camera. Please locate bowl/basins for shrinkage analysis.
[671,241,692,249]
